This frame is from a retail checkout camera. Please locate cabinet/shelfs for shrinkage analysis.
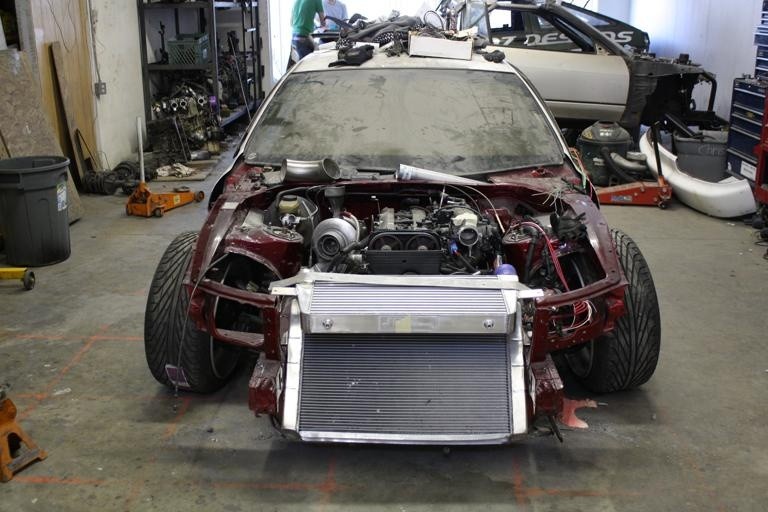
[135,1,260,143]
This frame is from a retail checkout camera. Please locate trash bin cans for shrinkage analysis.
[0,155,70,268]
